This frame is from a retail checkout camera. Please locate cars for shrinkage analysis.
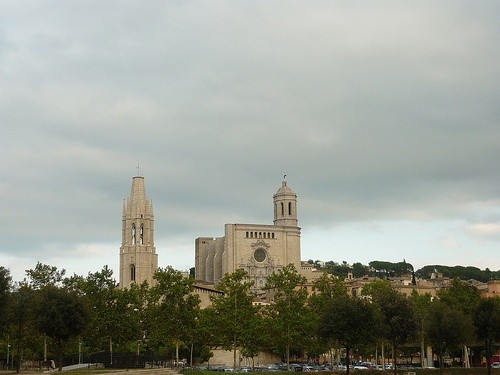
[177,361,441,375]
